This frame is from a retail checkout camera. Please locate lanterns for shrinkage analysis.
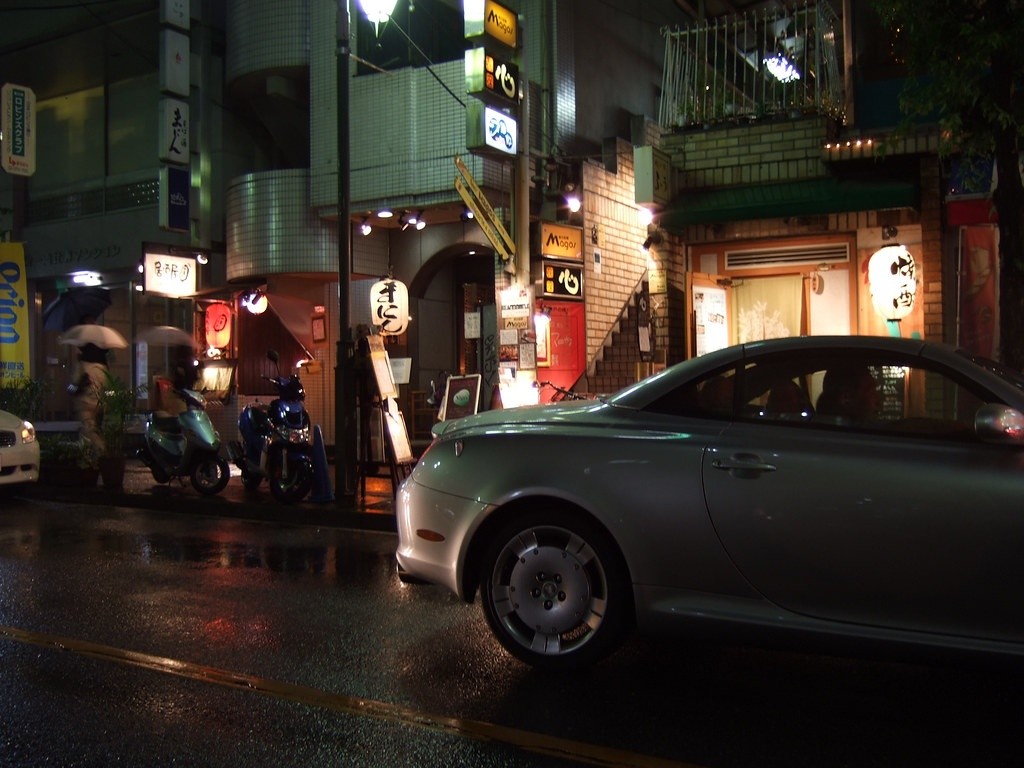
[369,278,409,337]
[203,304,231,349]
[867,244,919,329]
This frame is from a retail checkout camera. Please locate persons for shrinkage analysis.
[69,317,111,468]
[822,354,885,421]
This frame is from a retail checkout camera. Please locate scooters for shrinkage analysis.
[227,351,314,504]
[135,383,232,495]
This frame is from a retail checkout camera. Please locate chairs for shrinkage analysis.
[764,376,817,416]
[700,376,746,419]
[411,390,439,440]
[815,367,845,417]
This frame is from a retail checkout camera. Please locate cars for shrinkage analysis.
[0,407,40,492]
[392,333,1024,669]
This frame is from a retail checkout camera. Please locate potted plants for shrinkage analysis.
[36,367,153,495]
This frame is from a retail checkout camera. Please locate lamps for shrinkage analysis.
[399,209,426,232]
[460,204,474,223]
[361,213,372,236]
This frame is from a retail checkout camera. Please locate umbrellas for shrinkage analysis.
[133,325,199,347]
[42,287,112,330]
[58,324,129,349]
